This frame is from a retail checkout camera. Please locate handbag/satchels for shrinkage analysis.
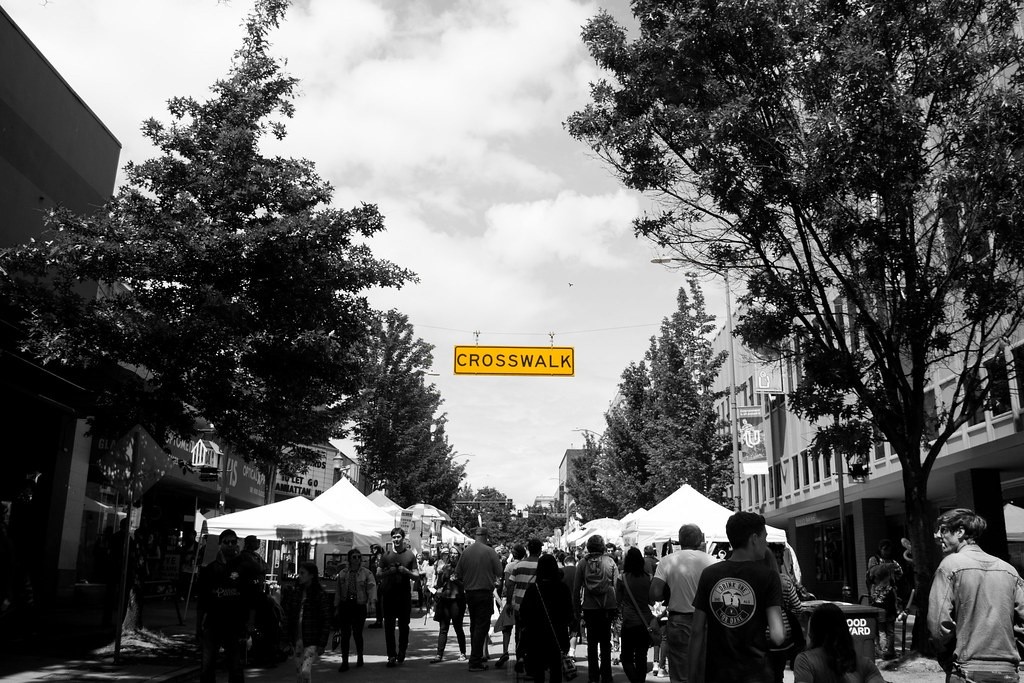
[563,656,579,681]
[332,621,340,651]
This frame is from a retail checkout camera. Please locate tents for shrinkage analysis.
[182,476,478,624]
[561,483,788,553]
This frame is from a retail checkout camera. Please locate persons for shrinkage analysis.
[89,509,1024,683]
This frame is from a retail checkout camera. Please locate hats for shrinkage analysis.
[679,526,701,546]
[587,535,605,552]
[476,527,487,534]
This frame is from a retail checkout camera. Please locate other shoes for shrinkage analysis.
[653,661,669,677]
[458,654,466,661]
[576,632,582,644]
[514,658,524,673]
[469,662,489,671]
[339,662,349,672]
[648,619,662,643]
[435,655,442,661]
[355,659,364,667]
[495,651,509,667]
[368,623,383,628]
[386,656,404,666]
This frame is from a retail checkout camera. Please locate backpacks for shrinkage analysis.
[584,554,610,593]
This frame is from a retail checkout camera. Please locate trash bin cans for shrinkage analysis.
[798,600,842,642]
[837,604,879,665]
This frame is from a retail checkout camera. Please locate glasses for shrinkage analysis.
[223,540,237,545]
[936,525,952,534]
[352,555,361,559]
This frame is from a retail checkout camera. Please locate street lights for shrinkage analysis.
[650,255,744,513]
[571,428,605,439]
